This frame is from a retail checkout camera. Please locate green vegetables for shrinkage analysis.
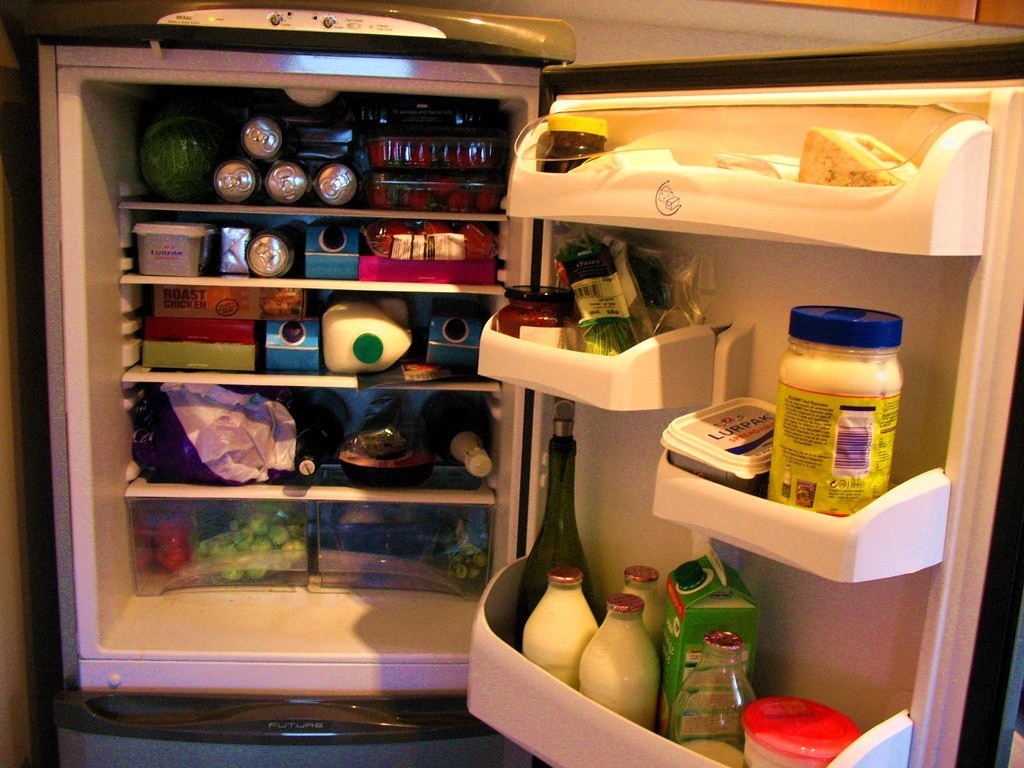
[555,233,672,356]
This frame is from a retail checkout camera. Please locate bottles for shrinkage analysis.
[291,388,350,477]
[514,418,593,651]
[419,391,494,477]
[578,593,660,733]
[669,629,756,768]
[521,566,598,690]
[617,565,665,653]
[322,299,416,377]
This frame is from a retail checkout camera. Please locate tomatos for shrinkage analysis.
[370,219,494,260]
[154,517,187,570]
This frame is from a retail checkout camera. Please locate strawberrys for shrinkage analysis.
[363,135,501,212]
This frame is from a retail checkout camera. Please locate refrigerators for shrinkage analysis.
[38,1,1024,768]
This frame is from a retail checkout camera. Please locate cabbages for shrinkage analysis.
[138,104,226,202]
[198,505,305,581]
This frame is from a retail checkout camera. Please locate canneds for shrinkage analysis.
[767,305,905,516]
[493,285,577,352]
[212,115,359,207]
[245,225,301,277]
